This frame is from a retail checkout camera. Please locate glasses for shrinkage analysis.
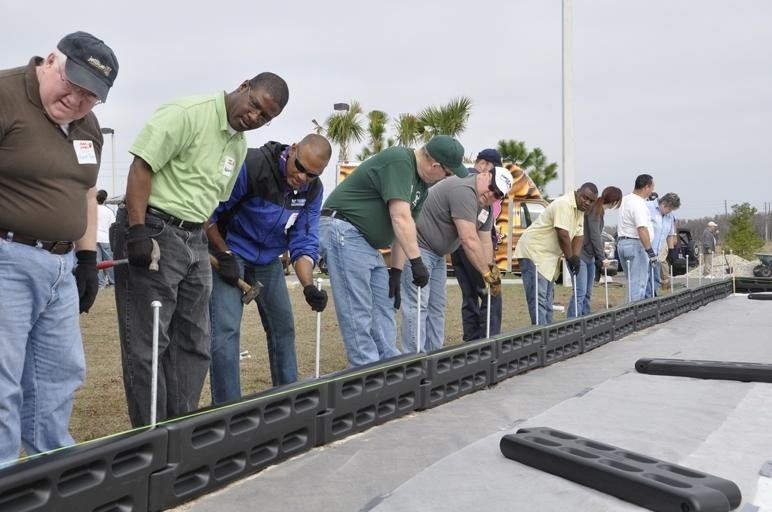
[246,86,271,128]
[294,146,320,179]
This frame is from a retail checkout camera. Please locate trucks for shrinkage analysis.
[335,156,563,279]
[597,230,620,280]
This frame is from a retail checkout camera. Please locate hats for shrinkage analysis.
[648,192,658,201]
[477,149,514,200]
[57,31,119,104]
[426,135,469,178]
[707,221,718,227]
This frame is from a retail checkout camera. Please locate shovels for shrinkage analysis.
[724,252,733,274]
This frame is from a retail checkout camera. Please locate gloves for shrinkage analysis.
[216,250,241,286]
[304,285,328,312]
[481,263,501,298]
[645,247,657,268]
[668,249,679,265]
[566,255,580,275]
[388,268,402,310]
[127,223,154,269]
[75,250,99,315]
[409,256,429,288]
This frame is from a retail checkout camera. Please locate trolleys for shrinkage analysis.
[752,253,772,277]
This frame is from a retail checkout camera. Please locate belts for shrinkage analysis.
[121,195,203,232]
[0,229,74,255]
[320,209,348,222]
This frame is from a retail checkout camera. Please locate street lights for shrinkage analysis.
[101,127,118,215]
[333,103,350,115]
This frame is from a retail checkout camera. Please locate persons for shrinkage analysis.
[451,148,502,341]
[400,166,513,353]
[616,174,679,303]
[207,134,332,406]
[566,185,622,319]
[317,135,468,368]
[109,73,290,429]
[512,182,598,326]
[96,190,116,289]
[702,222,718,276]
[0,33,120,464]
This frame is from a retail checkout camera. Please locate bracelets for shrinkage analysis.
[225,250,231,255]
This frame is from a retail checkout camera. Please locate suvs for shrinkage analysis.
[670,228,700,273]
